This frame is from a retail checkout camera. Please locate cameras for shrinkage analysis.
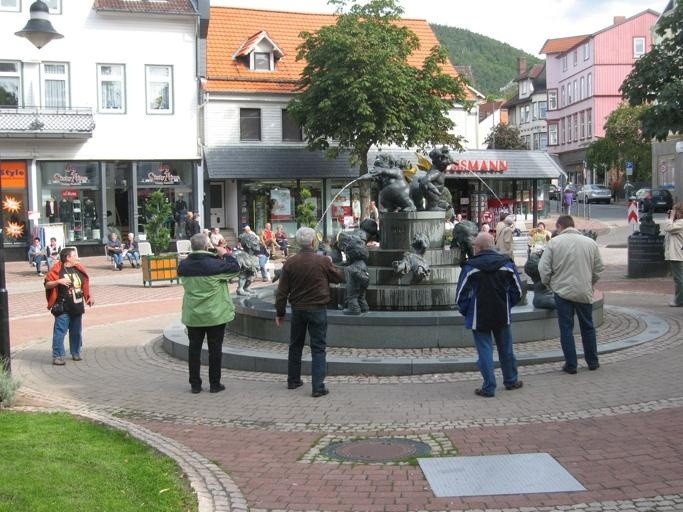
[667,209,671,214]
[207,248,217,253]
[75,290,84,299]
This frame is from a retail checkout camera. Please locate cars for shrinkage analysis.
[547,181,614,205]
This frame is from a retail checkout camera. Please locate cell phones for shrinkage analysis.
[323,251,327,256]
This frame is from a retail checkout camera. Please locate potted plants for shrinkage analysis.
[139,188,180,286]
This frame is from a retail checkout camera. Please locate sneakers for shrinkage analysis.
[53,357,66,365]
[311,389,330,397]
[210,384,224,393]
[288,379,303,389]
[475,388,495,397]
[505,381,524,389]
[73,355,81,360]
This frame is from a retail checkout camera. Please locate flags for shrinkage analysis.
[625,201,641,233]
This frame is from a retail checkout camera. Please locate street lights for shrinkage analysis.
[0,0,65,377]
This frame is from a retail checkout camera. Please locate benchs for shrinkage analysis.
[172,239,193,266]
[102,242,155,271]
[235,238,291,257]
[23,246,80,270]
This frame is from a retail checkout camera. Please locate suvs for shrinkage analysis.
[628,186,673,213]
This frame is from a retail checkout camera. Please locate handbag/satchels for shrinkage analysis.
[51,302,64,317]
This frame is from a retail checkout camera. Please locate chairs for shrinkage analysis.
[511,213,532,265]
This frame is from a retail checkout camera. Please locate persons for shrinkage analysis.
[44,247,94,365]
[272,226,342,397]
[142,192,188,227]
[174,232,241,393]
[27,237,45,277]
[604,173,636,202]
[453,230,524,398]
[329,137,476,314]
[662,203,682,306]
[365,189,573,275]
[106,232,140,271]
[538,213,605,372]
[165,209,297,297]
[271,192,360,218]
[44,237,59,271]
